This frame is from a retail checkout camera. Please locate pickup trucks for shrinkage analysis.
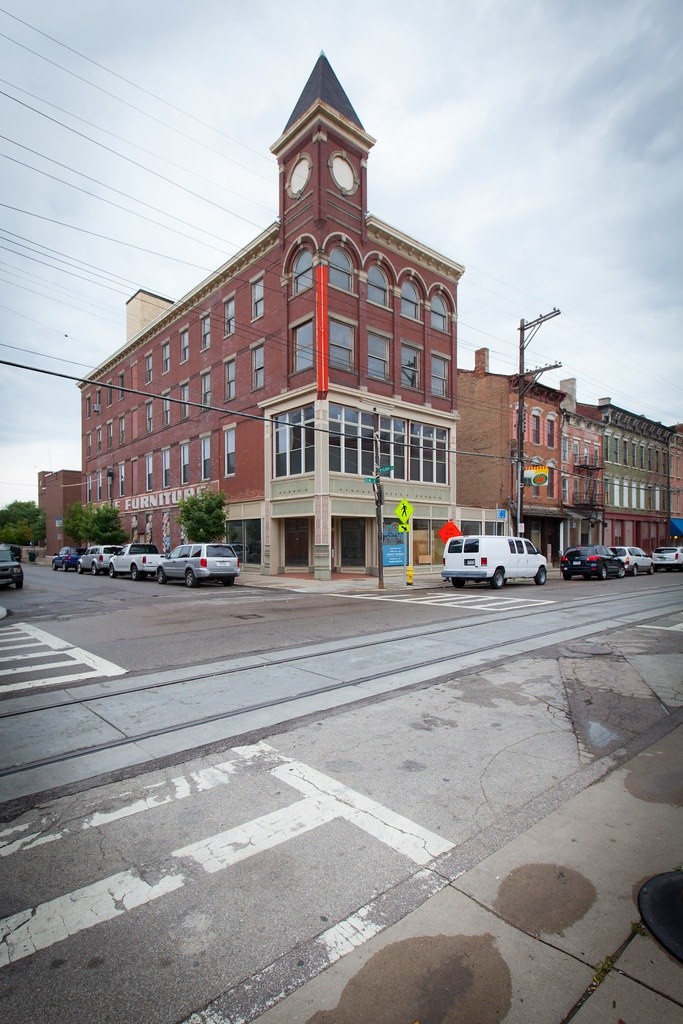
[106,543,166,581]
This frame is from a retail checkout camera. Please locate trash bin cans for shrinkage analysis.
[28,552,36,562]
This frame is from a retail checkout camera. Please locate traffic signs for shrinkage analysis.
[377,466,395,473]
[364,476,378,484]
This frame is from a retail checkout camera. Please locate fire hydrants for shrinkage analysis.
[404,563,416,585]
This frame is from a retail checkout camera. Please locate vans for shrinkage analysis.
[439,533,549,590]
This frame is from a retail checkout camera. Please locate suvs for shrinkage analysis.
[0,546,24,590]
[609,547,656,576]
[651,546,683,572]
[156,543,242,588]
[50,546,88,572]
[560,545,628,582]
[75,544,125,577]
